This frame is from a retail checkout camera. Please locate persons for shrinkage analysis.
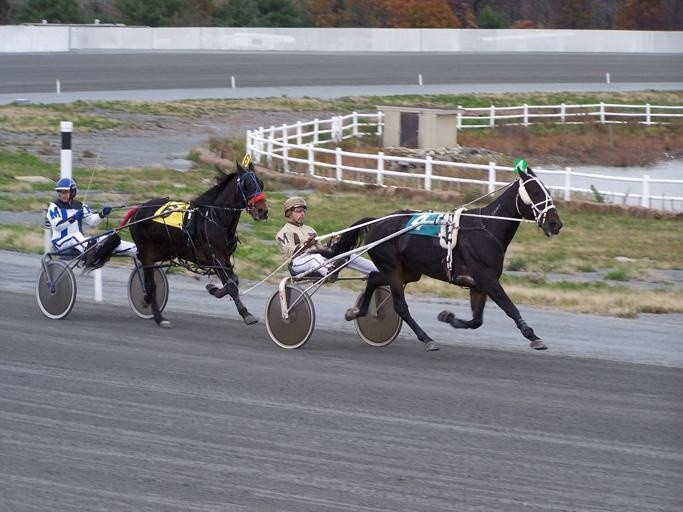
[275,196,380,283]
[46,177,139,260]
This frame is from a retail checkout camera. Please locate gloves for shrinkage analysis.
[102,206,111,216]
[303,236,318,250]
[73,207,83,222]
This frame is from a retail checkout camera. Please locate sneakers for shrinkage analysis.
[327,258,345,283]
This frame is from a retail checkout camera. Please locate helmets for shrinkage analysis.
[54,178,76,201]
[283,196,307,212]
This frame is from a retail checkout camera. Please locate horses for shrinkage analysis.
[76,160,270,330]
[306,164,564,354]
